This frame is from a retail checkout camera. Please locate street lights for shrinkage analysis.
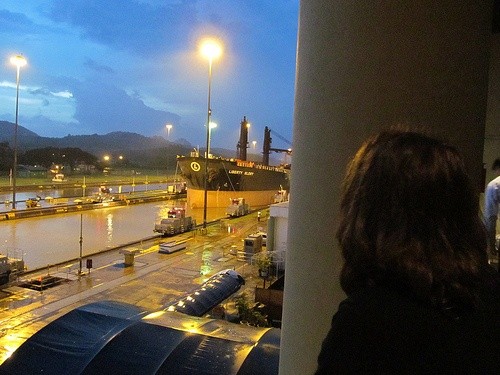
[7,54,28,209]
[197,38,221,229]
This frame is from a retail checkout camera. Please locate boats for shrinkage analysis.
[175,115,295,226]
[52,173,68,182]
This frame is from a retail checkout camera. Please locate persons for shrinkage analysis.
[311,119,500,375]
[482,156,500,274]
[257,209,262,222]
